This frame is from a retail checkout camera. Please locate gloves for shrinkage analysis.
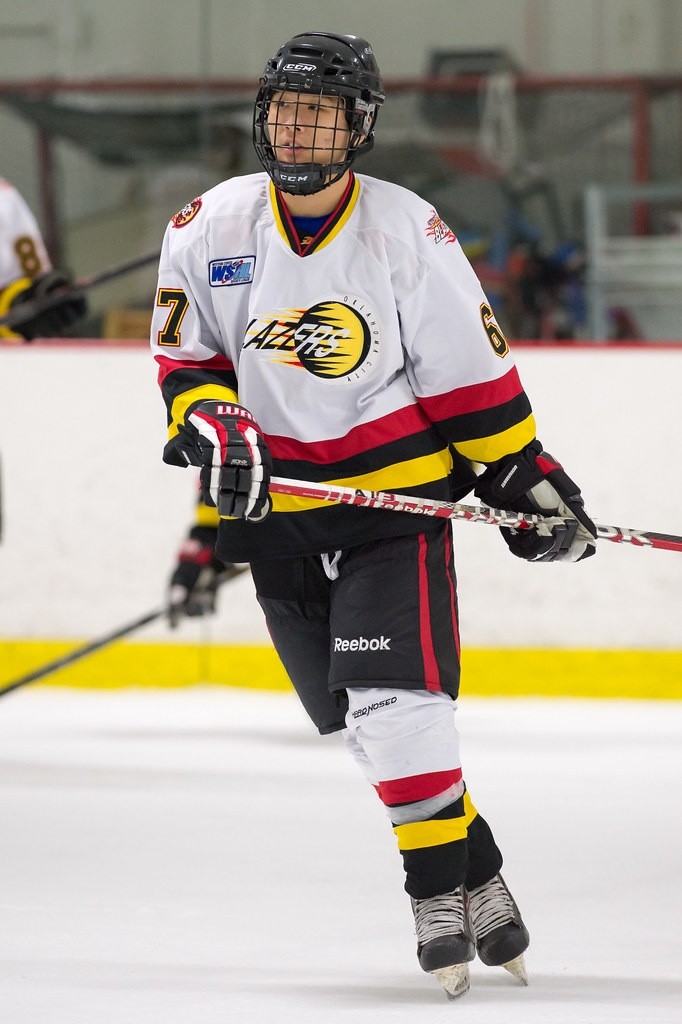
[168,526,231,629]
[476,440,598,564]
[8,274,85,340]
[176,399,273,524]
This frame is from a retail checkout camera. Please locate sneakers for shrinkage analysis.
[465,873,533,987]
[410,882,475,999]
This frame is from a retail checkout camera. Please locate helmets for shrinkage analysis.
[252,32,385,198]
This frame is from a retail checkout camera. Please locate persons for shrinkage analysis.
[0,174,90,544]
[149,31,597,1002]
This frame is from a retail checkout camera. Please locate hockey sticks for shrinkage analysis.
[266,474,682,560]
[1,560,249,702]
